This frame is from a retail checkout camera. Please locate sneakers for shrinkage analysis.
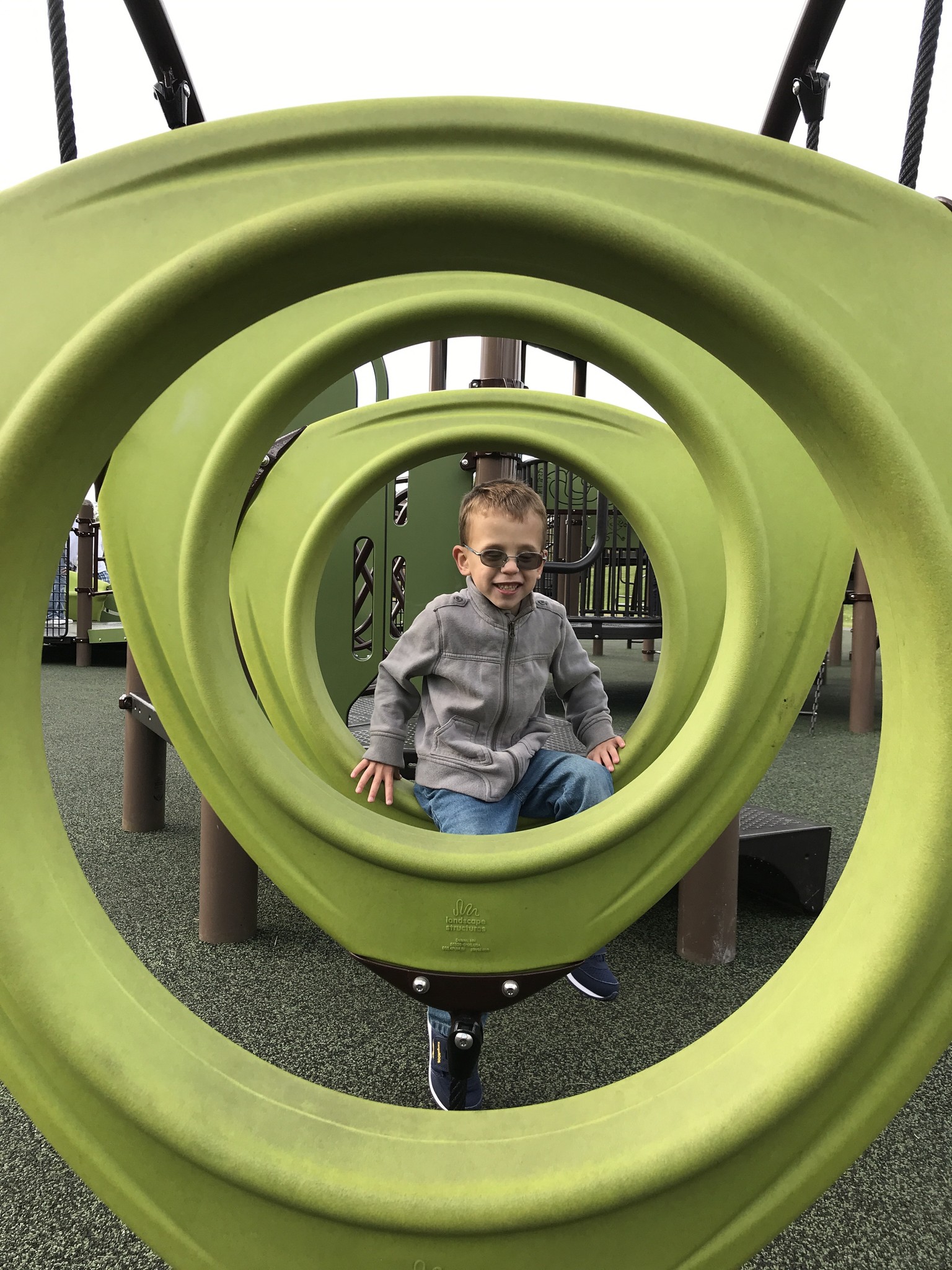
[565,946,620,1002]
[428,1012,482,1111]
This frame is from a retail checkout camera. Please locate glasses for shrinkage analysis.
[462,542,546,570]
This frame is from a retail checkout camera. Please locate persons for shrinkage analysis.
[351,478,626,1109]
